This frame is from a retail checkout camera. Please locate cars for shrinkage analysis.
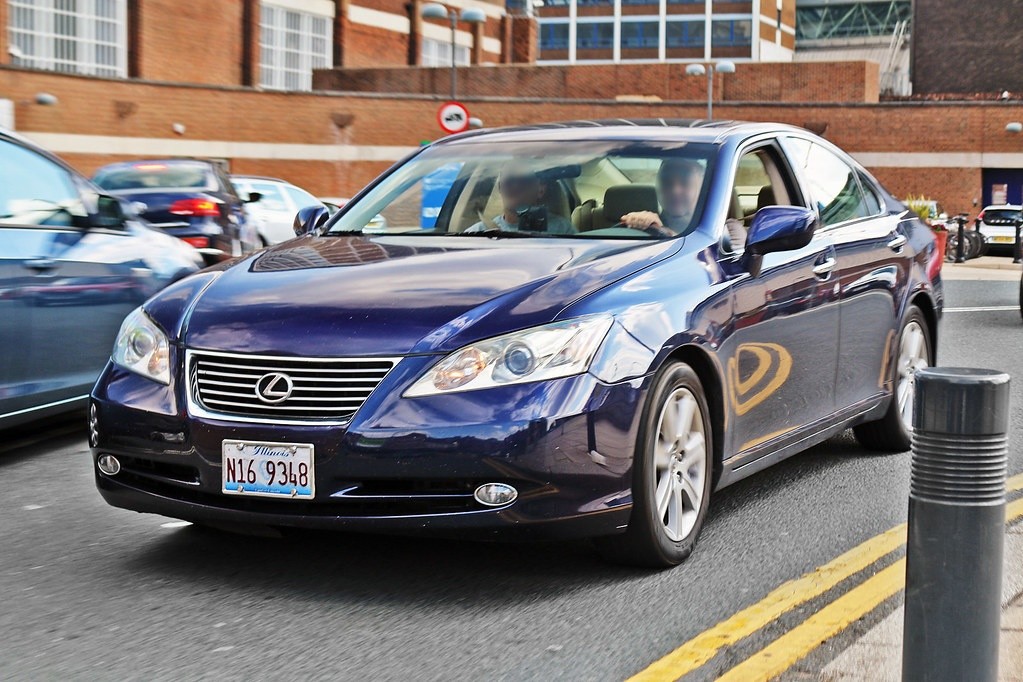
[972,204,1023,256]
[87,114,943,569]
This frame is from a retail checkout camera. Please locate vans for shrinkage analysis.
[0,132,205,431]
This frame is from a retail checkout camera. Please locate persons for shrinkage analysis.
[463,159,578,234]
[621,159,748,255]
